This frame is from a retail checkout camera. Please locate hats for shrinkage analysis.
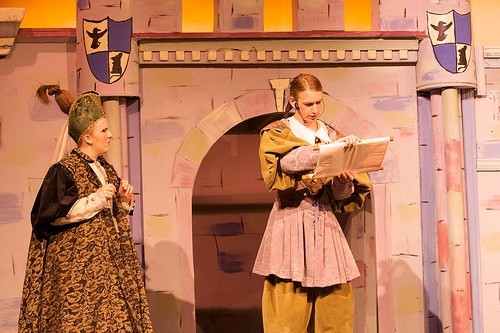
[68,94,106,143]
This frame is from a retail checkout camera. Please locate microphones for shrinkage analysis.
[295,103,307,123]
